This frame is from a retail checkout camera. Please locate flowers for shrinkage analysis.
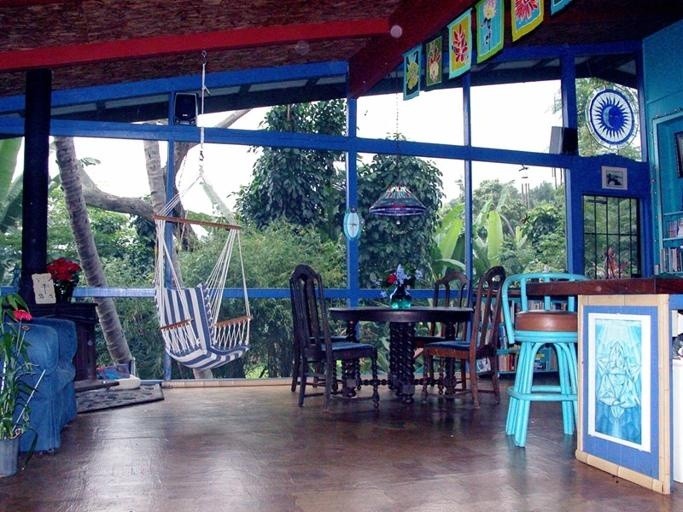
[47,258,81,284]
[0,292,38,469]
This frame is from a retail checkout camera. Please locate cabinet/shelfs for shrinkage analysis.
[652,111,683,274]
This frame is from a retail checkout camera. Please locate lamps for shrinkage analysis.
[368,68,425,215]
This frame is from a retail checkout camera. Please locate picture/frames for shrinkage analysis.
[602,165,628,190]
[673,131,683,177]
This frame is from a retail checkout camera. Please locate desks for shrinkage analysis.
[34,302,99,380]
[526,278,683,496]
[328,304,478,410]
[478,287,573,380]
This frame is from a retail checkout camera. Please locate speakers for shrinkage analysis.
[173,93,198,127]
[549,126,575,155]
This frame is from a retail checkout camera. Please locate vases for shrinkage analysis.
[54,281,74,302]
[0,439,18,477]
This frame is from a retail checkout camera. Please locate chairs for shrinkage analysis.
[385,264,505,410]
[290,264,380,410]
[0,287,79,456]
[496,272,584,443]
